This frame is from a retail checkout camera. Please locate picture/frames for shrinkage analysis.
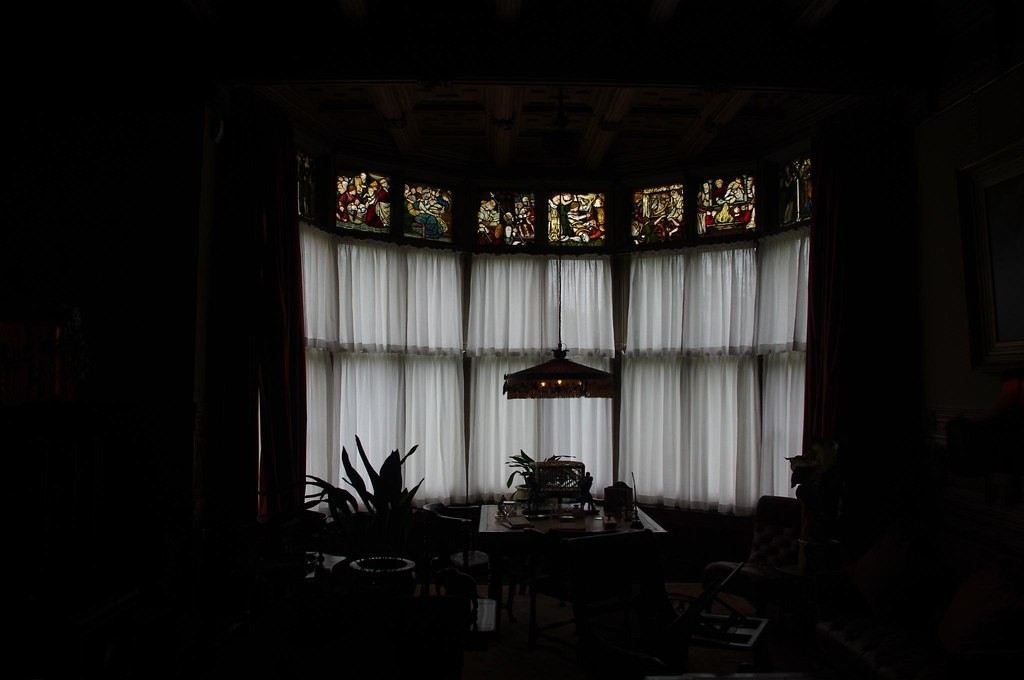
[954,136,1024,377]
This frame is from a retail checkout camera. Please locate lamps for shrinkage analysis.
[502,191,616,400]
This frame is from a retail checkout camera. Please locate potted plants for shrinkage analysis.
[506,451,570,520]
[300,436,423,601]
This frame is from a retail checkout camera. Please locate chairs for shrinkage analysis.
[703,496,801,615]
[438,506,516,630]
[524,529,618,668]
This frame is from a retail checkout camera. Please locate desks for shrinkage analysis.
[477,504,668,632]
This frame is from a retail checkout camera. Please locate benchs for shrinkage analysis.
[816,621,946,680]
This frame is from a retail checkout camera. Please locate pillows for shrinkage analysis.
[842,518,923,619]
[935,566,1024,670]
